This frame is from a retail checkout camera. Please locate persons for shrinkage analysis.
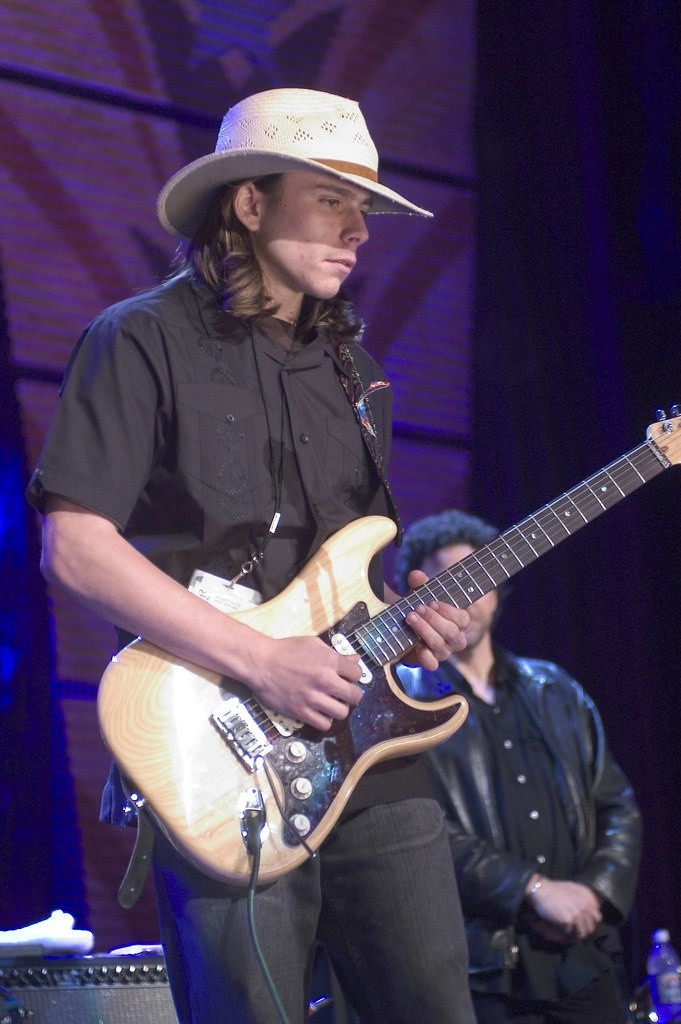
[28,88,477,1024]
[397,510,641,1024]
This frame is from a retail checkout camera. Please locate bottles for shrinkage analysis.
[646,931,681,1024]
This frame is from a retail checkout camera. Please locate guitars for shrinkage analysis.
[95,406,680,887]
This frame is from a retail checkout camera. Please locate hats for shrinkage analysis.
[156,86,433,240]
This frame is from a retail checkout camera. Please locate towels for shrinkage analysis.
[0,909,95,959]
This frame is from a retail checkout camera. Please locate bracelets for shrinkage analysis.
[527,874,544,898]
